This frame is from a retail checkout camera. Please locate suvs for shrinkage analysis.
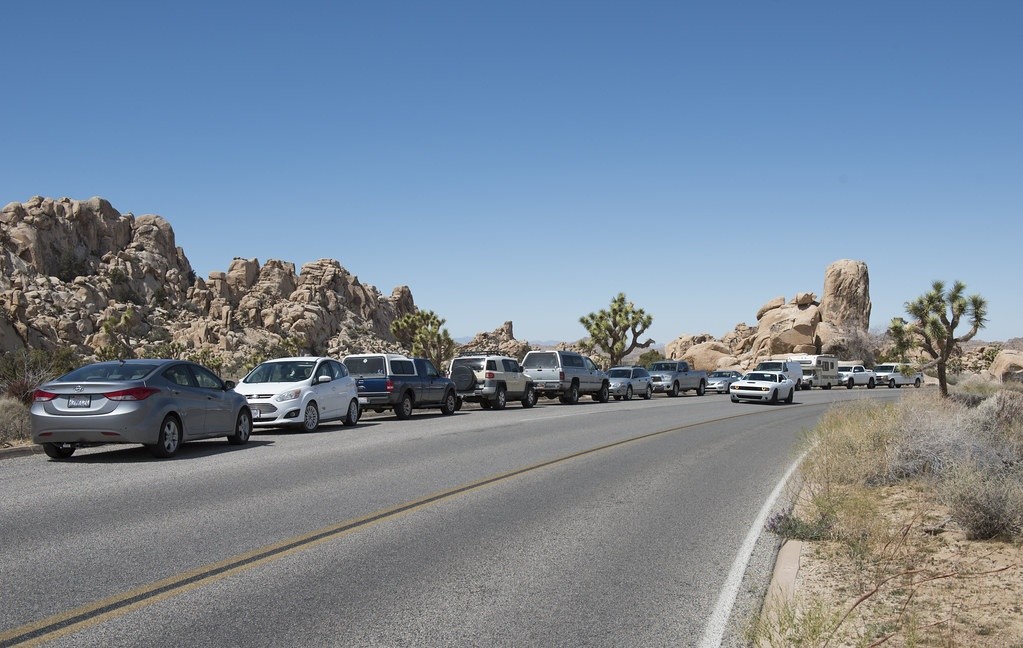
[520,350,610,405]
[444,350,537,411]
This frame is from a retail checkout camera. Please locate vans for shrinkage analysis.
[752,360,803,391]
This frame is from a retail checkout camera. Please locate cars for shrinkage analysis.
[592,365,653,400]
[729,369,795,405]
[232,356,360,434]
[30,358,254,459]
[704,370,743,394]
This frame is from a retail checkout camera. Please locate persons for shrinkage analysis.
[769,375,776,382]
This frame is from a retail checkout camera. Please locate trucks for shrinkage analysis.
[788,354,838,390]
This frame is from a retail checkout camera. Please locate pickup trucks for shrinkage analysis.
[873,363,923,388]
[647,360,708,397]
[337,353,457,420]
[838,364,876,390]
